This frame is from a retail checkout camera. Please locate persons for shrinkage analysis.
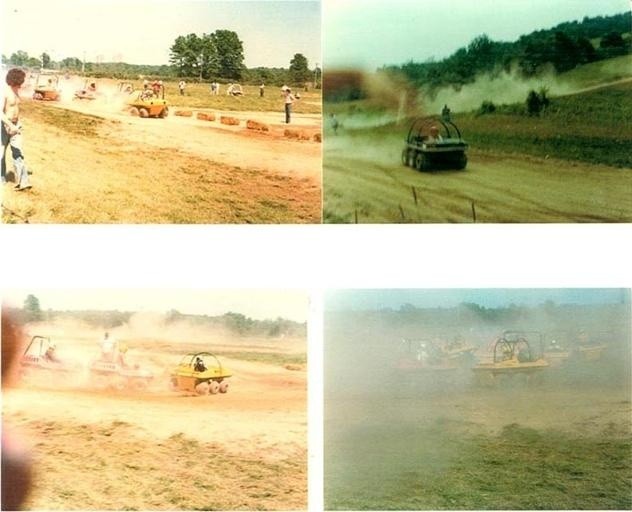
[1,306,37,511]
[1,69,35,190]
[194,358,210,375]
[178,78,189,94]
[280,82,301,126]
[329,111,339,134]
[257,82,267,98]
[88,82,96,91]
[208,80,242,96]
[46,342,57,362]
[135,80,169,101]
[118,341,136,366]
[428,126,444,144]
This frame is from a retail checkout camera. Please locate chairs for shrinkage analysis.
[194,359,205,371]
